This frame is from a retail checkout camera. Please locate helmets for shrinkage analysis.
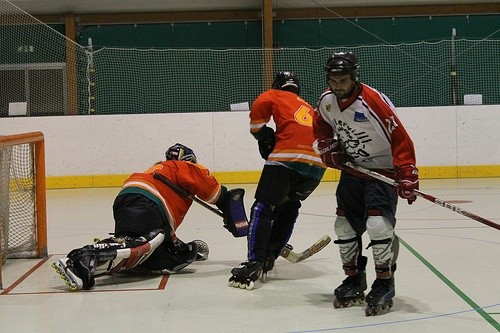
[165,143,197,164]
[324,51,359,80]
[272,72,301,92]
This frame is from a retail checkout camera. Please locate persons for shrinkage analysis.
[228,72,326,291]
[312,51,419,315]
[52,142,248,290]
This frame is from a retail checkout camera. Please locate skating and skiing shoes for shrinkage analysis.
[364,262,397,316]
[52,244,97,291]
[229,252,275,291]
[333,256,368,308]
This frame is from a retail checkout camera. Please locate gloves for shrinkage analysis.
[317,139,343,167]
[257,130,275,159]
[394,167,421,203]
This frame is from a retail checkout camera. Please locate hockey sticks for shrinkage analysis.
[150,174,332,264]
[344,162,500,233]
